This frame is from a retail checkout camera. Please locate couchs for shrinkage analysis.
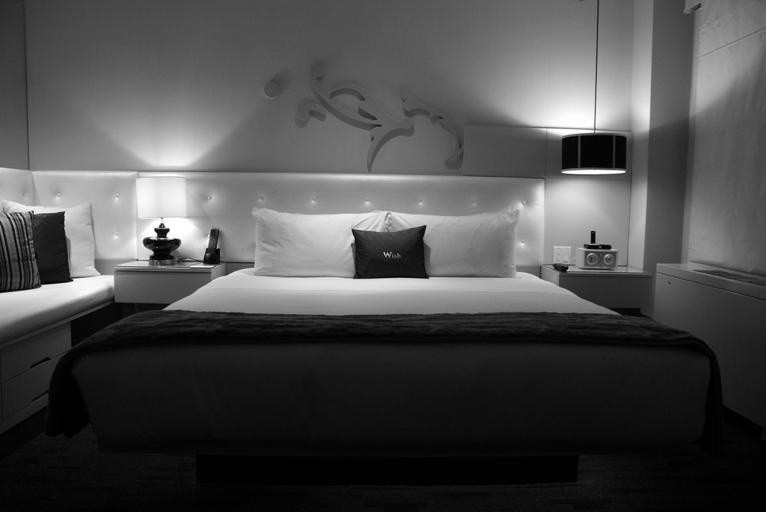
[2,167,250,337]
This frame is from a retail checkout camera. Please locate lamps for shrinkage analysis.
[135,176,187,265]
[560,1,628,175]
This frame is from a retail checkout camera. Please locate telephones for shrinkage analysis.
[204,229,220,264]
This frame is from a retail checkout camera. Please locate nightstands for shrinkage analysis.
[538,264,651,318]
[114,261,230,317]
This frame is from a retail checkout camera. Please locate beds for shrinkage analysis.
[48,173,723,497]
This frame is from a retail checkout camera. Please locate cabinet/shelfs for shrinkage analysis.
[1,323,73,432]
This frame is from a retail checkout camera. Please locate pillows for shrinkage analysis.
[252,207,387,277]
[389,208,521,277]
[33,211,71,284]
[1,210,45,292]
[3,200,102,277]
[349,224,428,280]
